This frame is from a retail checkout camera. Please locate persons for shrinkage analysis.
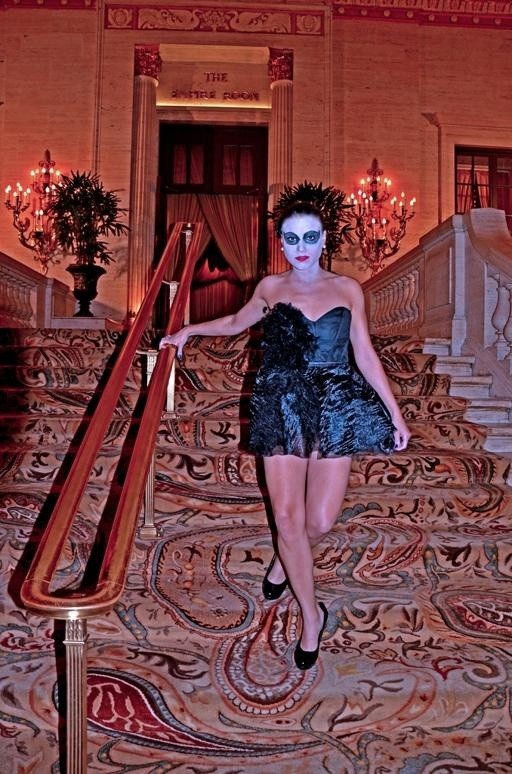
[156,180,412,670]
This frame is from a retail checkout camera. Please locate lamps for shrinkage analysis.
[4,150,70,277]
[346,157,417,277]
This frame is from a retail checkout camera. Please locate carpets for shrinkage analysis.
[0,322,512,773]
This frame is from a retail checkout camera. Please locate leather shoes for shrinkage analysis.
[261,548,288,600]
[293,601,329,669]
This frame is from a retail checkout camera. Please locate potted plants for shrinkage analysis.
[47,166,135,318]
